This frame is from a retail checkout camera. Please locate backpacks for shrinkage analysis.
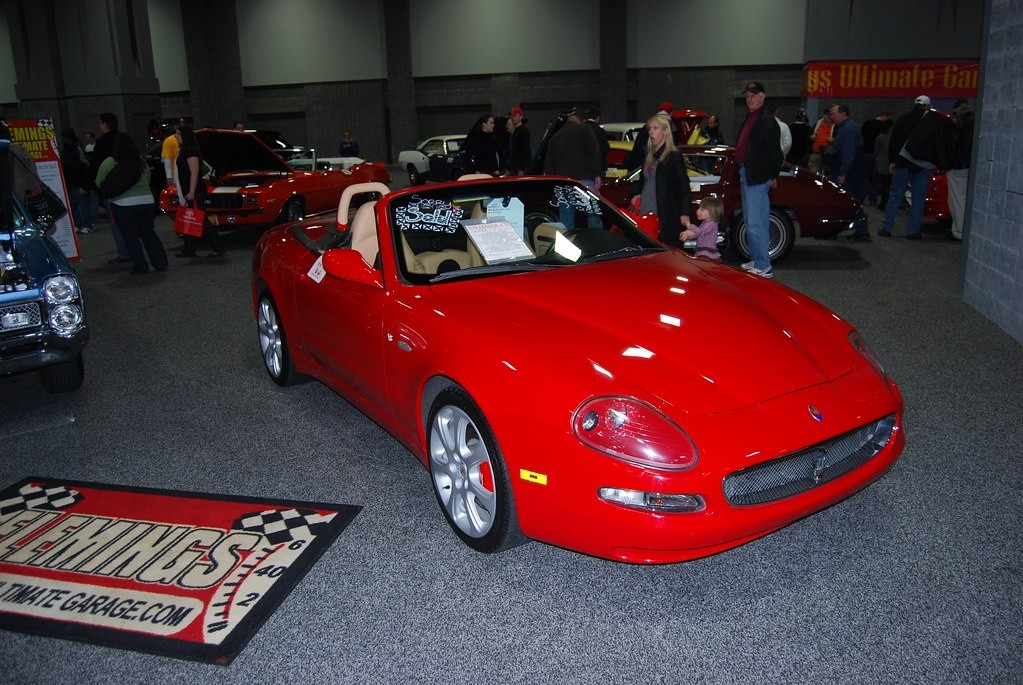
[905,109,954,165]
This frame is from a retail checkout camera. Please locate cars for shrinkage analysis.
[0,139,90,394]
[599,108,868,266]
[905,108,957,227]
[398,134,500,187]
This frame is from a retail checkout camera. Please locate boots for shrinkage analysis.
[845,218,870,241]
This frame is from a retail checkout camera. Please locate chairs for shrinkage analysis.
[466,195,539,267]
[337,183,426,277]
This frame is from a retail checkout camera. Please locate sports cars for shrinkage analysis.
[250,174,905,565]
[159,128,390,241]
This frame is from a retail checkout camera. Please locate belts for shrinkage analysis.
[738,163,745,168]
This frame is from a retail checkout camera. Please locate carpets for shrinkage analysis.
[0,476,364,668]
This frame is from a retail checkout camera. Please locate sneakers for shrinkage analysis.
[741,260,755,270]
[747,266,773,279]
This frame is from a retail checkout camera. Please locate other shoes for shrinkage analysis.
[209,250,225,259]
[945,229,962,241]
[907,233,921,240]
[74,227,79,232]
[81,223,96,234]
[109,256,132,264]
[175,250,195,258]
[878,228,891,236]
[129,265,147,275]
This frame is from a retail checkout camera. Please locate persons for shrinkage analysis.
[543,106,610,232]
[830,103,864,193]
[944,99,974,242]
[0,117,38,213]
[59,126,111,235]
[149,117,226,258]
[233,120,246,130]
[808,108,838,175]
[79,111,140,264]
[621,101,693,250]
[95,131,168,275]
[469,106,531,177]
[876,92,945,241]
[860,108,893,206]
[678,195,725,263]
[338,129,363,159]
[701,113,725,145]
[733,79,793,279]
[789,106,814,172]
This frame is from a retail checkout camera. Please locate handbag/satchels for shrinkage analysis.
[843,163,878,201]
[98,157,142,199]
[24,185,67,226]
[175,196,205,238]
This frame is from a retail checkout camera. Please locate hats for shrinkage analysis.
[508,107,523,116]
[560,108,576,117]
[915,95,930,105]
[742,82,764,94]
[794,108,808,119]
[659,102,672,114]
